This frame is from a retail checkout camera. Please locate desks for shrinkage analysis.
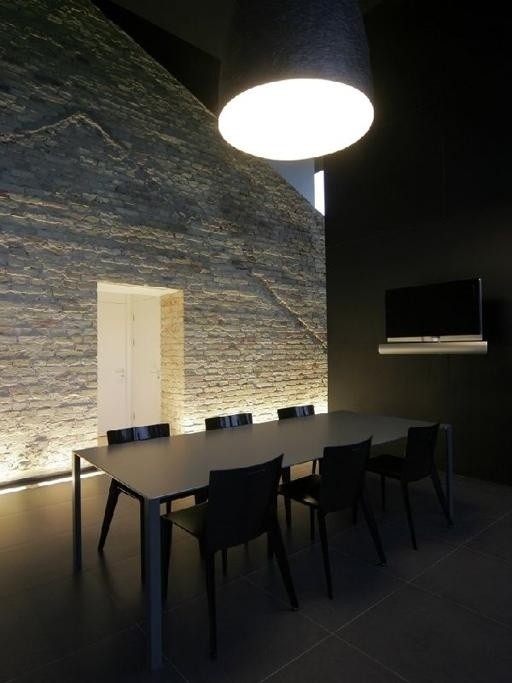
[70,410,454,671]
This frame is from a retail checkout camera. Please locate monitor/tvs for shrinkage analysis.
[385,277,484,342]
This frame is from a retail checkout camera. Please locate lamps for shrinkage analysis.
[216,0,377,162]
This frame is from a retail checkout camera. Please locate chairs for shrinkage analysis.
[160,453,301,657]
[97,422,174,557]
[365,422,455,551]
[194,412,252,565]
[276,431,388,600]
[277,406,317,526]
